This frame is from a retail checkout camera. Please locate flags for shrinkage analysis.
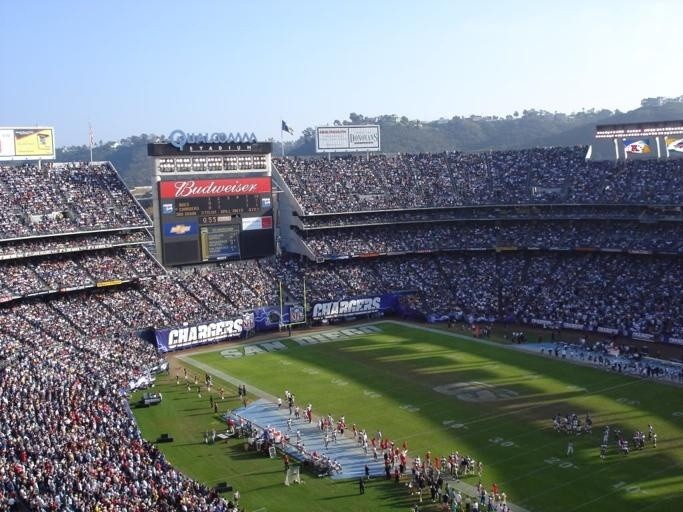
[281,119,295,135]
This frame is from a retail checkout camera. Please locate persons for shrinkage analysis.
[275,144,683,383]
[1,161,298,512]
[599,424,657,459]
[552,411,593,455]
[284,390,510,512]
